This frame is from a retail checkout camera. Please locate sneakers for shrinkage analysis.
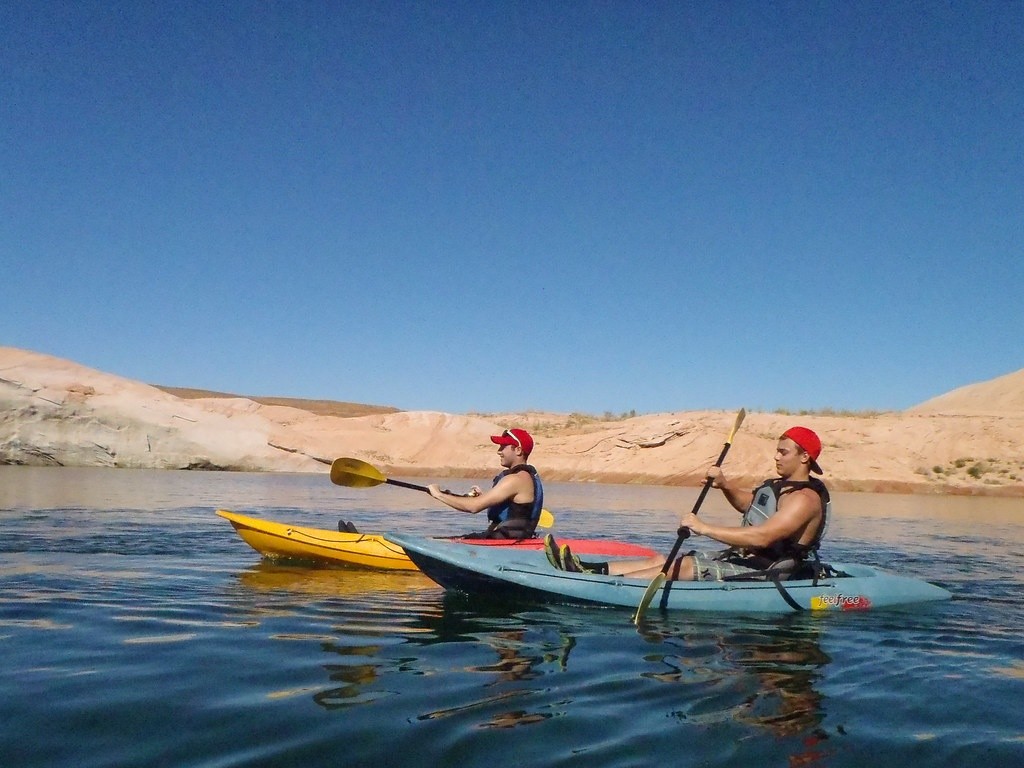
[544,533,594,574]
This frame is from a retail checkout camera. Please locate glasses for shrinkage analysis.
[502,429,521,447]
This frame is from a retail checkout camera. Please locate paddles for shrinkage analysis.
[329,457,554,528]
[634,406,748,613]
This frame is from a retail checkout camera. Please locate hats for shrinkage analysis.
[784,426,824,475]
[491,428,534,455]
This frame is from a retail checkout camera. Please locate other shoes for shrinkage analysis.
[339,519,358,534]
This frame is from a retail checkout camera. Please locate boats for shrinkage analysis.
[215,507,660,573]
[383,527,956,612]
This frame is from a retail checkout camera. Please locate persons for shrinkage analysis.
[544,427,831,581]
[427,429,544,539]
